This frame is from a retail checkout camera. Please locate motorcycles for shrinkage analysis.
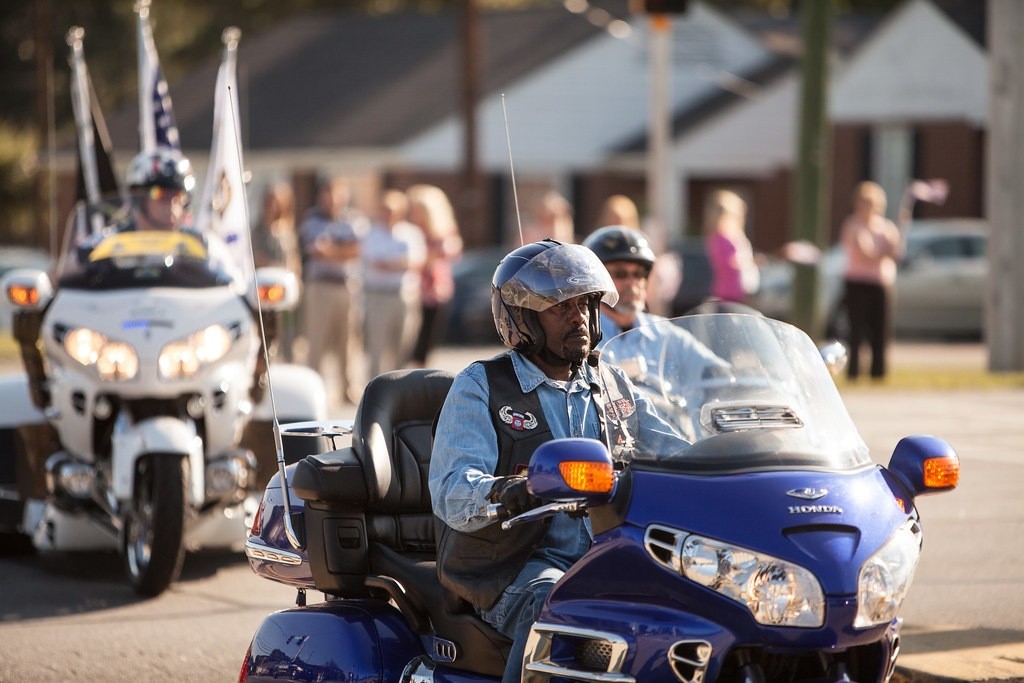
[233,311,960,682]
[0,199,303,601]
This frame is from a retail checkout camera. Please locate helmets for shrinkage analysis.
[125,146,193,207]
[584,229,654,276]
[490,239,601,352]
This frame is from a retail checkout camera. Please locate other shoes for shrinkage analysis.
[339,388,358,409]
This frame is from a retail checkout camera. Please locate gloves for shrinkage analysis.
[488,477,556,525]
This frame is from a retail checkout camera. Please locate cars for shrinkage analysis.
[754,215,989,338]
[427,245,505,350]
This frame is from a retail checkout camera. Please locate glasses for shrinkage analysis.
[140,187,190,205]
[608,269,648,282]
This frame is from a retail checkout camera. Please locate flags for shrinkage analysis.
[66,0,255,295]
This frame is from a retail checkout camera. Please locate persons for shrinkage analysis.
[60,145,233,292]
[428,240,693,683]
[252,176,906,434]
